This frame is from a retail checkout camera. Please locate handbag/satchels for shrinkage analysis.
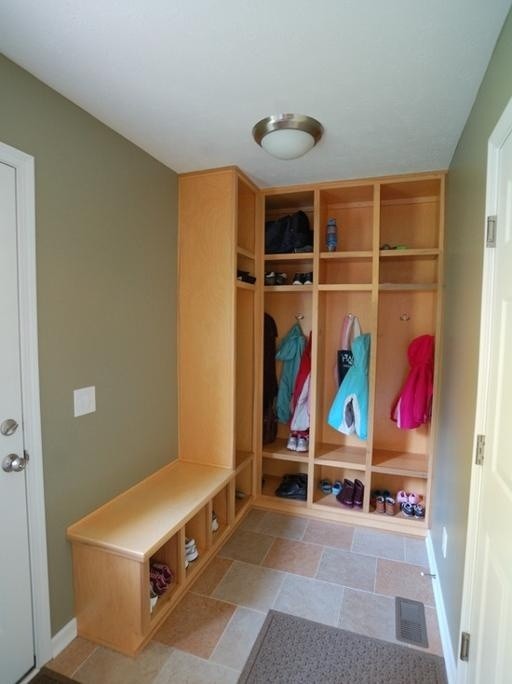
[264,210,309,253]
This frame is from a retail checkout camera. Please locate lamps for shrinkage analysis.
[251,113,325,162]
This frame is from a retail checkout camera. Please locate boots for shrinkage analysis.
[318,479,364,508]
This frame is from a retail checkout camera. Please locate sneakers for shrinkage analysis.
[371,490,424,519]
[149,558,175,613]
[264,271,313,285]
[184,536,198,568]
[286,431,309,452]
[212,512,218,531]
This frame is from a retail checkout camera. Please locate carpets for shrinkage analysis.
[236,607,449,684]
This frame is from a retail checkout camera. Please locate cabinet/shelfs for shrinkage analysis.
[256,172,445,537]
[67,458,232,658]
[174,162,256,532]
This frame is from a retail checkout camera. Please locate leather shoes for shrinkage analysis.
[236,490,246,499]
[275,474,307,500]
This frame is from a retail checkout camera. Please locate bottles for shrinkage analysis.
[325,215,337,252]
